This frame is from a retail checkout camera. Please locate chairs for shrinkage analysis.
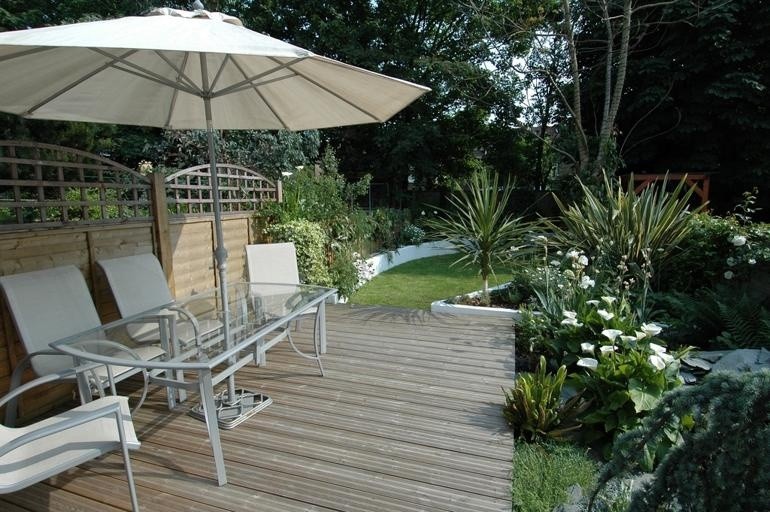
[0,363,141,512]
[244,241,326,367]
[95,252,225,404]
[0,264,177,460]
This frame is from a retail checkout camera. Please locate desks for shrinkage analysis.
[48,281,340,487]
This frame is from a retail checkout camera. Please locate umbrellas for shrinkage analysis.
[0,1,432,404]
